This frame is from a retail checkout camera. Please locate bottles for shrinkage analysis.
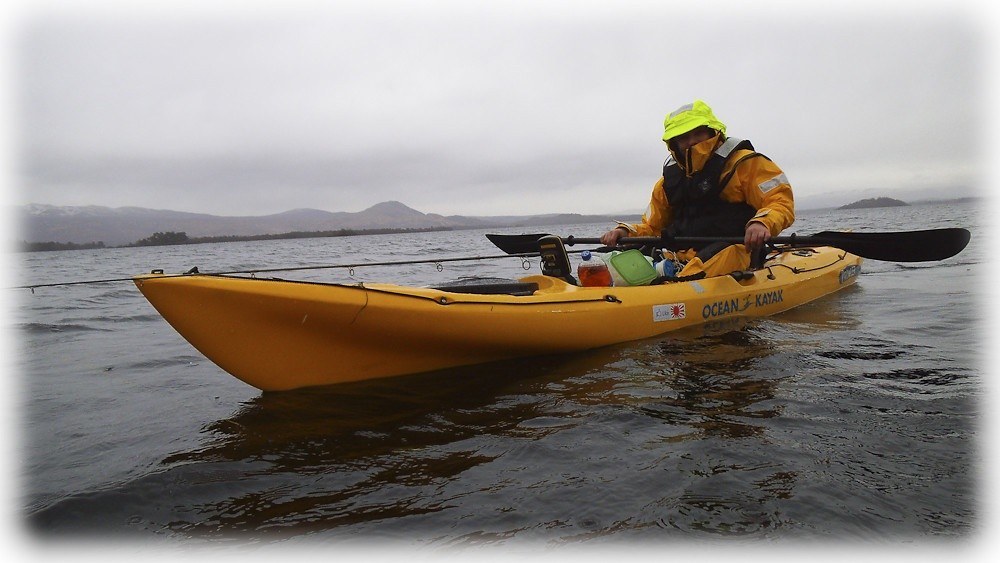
[577,251,614,287]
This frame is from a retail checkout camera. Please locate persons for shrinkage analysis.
[600,101,796,278]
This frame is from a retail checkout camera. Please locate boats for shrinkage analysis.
[132,227,862,394]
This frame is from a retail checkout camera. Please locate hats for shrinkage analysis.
[662,99,726,151]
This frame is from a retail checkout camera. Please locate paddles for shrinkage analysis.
[484,224,972,264]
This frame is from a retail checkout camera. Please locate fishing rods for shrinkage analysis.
[4,238,681,298]
